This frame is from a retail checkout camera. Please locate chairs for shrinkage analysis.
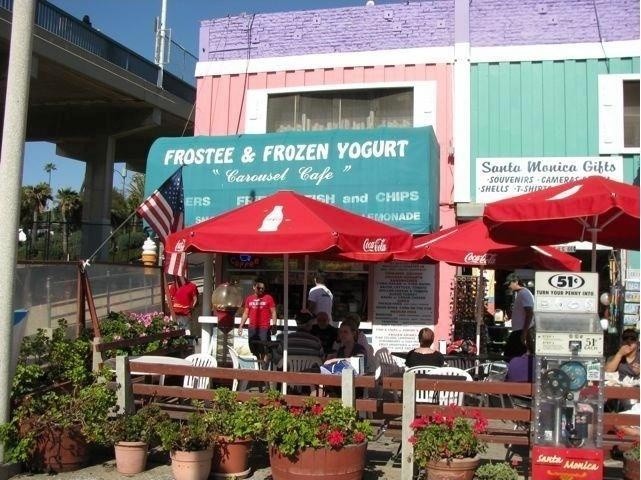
[105,340,530,406]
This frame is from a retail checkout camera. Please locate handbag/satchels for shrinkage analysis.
[320,360,358,375]
[447,339,477,356]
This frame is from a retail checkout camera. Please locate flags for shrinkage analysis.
[133,164,188,281]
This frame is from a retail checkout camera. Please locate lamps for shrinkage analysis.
[209,282,243,333]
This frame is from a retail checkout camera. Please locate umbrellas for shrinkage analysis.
[164,189,415,395]
[340,215,583,378]
[483,173,639,255]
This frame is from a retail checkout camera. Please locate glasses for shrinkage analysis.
[622,335,637,341]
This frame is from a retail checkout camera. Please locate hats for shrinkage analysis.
[294,309,317,324]
[503,273,520,286]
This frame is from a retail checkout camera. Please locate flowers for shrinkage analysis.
[265,391,373,456]
[408,403,488,465]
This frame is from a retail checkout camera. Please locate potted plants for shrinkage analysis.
[150,411,214,479]
[0,385,117,470]
[80,404,170,476]
[188,388,263,479]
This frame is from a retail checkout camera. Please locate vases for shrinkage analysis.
[426,456,480,479]
[268,443,368,480]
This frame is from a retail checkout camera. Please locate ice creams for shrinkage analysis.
[141,237,157,275]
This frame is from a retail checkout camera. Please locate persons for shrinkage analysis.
[161,274,200,328]
[236,271,368,393]
[605,325,640,381]
[403,327,446,370]
[501,271,536,382]
